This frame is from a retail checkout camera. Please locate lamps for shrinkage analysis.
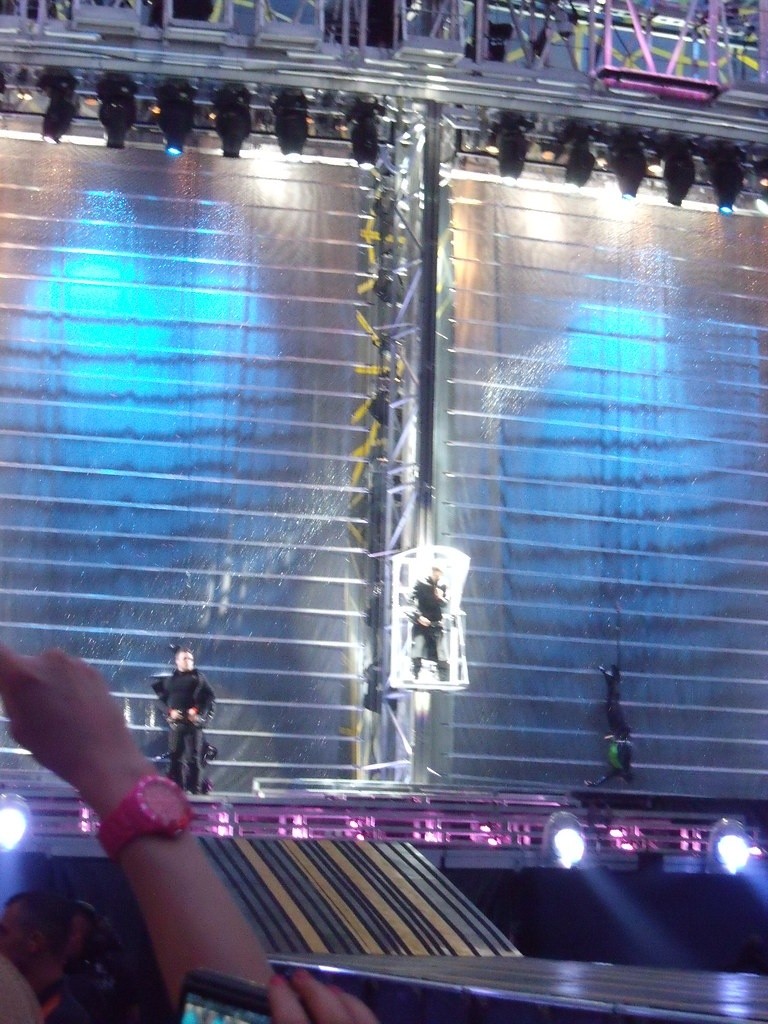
[606,128,657,196]
[658,137,706,206]
[268,88,314,156]
[492,111,534,181]
[149,76,199,158]
[552,121,604,186]
[710,144,749,217]
[30,72,80,142]
[95,72,138,149]
[213,81,252,158]
[343,96,387,165]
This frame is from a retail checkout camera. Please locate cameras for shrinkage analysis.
[176,971,276,1024]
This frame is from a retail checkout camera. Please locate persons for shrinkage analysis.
[0,644,379,1024]
[404,563,451,681]
[156,646,216,795]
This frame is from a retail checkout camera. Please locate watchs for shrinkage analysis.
[94,774,198,857]
